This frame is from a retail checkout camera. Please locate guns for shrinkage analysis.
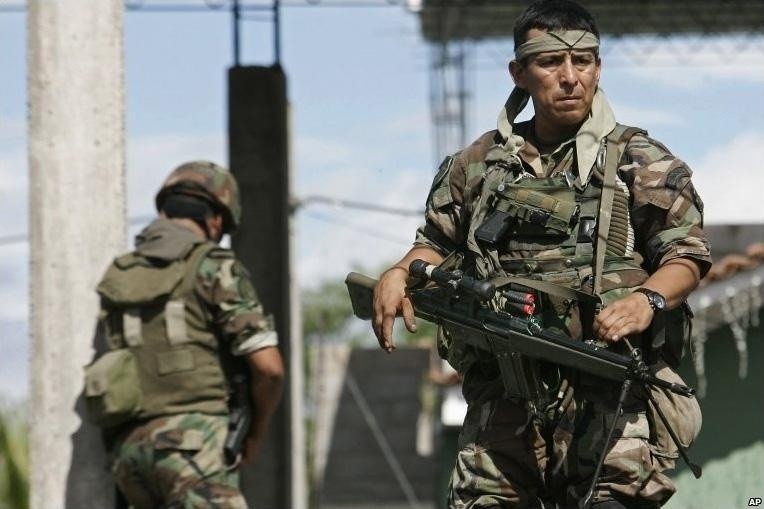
[344,270,702,506]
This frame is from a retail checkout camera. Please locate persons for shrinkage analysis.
[369,2,715,509]
[79,161,285,508]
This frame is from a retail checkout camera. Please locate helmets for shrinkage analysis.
[155,159,245,231]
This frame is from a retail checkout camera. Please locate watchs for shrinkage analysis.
[632,287,668,318]
